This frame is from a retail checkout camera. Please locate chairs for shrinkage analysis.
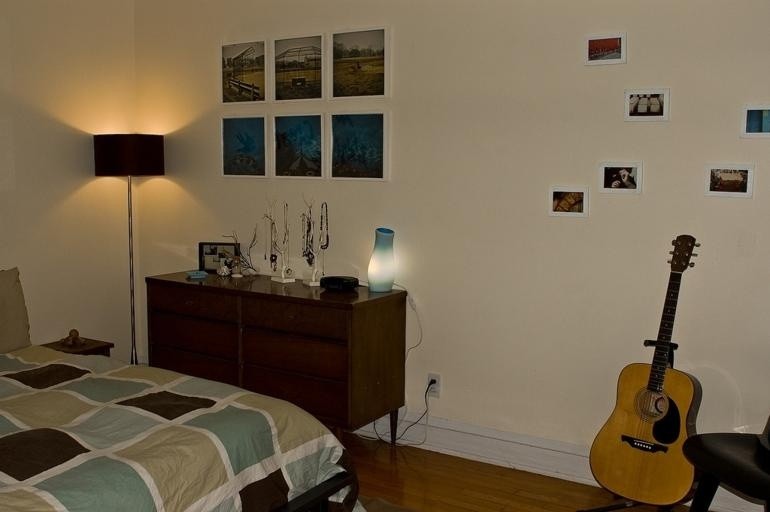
[674,408,770,511]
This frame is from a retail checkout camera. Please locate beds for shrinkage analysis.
[1,263,364,509]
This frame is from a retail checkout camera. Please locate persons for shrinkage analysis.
[610,168,638,189]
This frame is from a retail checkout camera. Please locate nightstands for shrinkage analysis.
[38,329,115,360]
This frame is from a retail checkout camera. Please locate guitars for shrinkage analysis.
[588,234,702,506]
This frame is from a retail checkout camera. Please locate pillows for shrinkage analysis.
[3,266,33,355]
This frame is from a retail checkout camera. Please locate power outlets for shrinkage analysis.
[427,372,440,393]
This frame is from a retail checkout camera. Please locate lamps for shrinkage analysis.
[366,227,398,292]
[90,130,165,366]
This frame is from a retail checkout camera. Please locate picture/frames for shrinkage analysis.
[197,241,241,274]
[598,160,644,194]
[217,26,391,184]
[704,161,754,200]
[582,32,626,66]
[739,104,770,139]
[548,184,590,219]
[623,88,670,125]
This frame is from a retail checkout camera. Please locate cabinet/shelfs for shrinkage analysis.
[138,269,410,473]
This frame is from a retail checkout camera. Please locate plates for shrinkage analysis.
[187,271,208,279]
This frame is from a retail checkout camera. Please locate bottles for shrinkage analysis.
[232,256,240,274]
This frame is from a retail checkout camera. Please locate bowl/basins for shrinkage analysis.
[320,290,359,303]
[321,276,359,291]
